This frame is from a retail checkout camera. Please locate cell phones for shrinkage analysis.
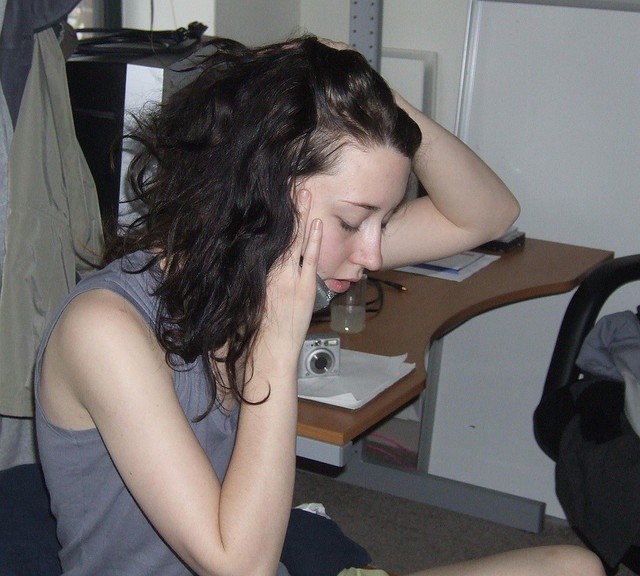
[293,255,334,314]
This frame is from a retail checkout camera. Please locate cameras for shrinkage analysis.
[299,331,342,378]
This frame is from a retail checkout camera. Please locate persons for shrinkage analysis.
[31,31,606,576]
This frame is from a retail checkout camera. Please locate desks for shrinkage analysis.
[296,237,615,468]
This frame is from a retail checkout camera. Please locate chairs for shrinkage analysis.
[533,253,640,575]
[1,463,373,575]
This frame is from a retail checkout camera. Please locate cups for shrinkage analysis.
[327,274,369,336]
